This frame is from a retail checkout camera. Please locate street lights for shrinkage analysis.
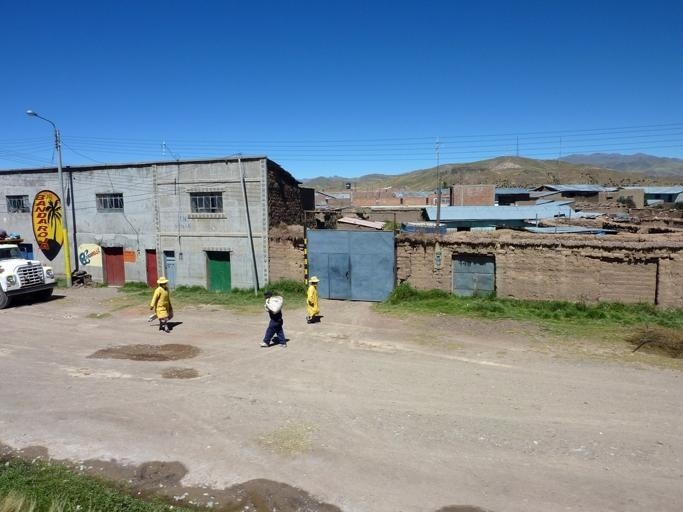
[26,109,73,288]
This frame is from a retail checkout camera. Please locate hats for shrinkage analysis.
[264,291,272,297]
[156,277,169,284]
[308,276,320,283]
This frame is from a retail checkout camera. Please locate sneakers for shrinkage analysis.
[279,343,287,347]
[158,325,170,333]
[260,342,268,347]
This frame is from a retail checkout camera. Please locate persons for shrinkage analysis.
[306,276,320,323]
[150,277,173,333]
[261,291,286,347]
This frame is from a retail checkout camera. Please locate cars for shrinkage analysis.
[0,242,57,308]
